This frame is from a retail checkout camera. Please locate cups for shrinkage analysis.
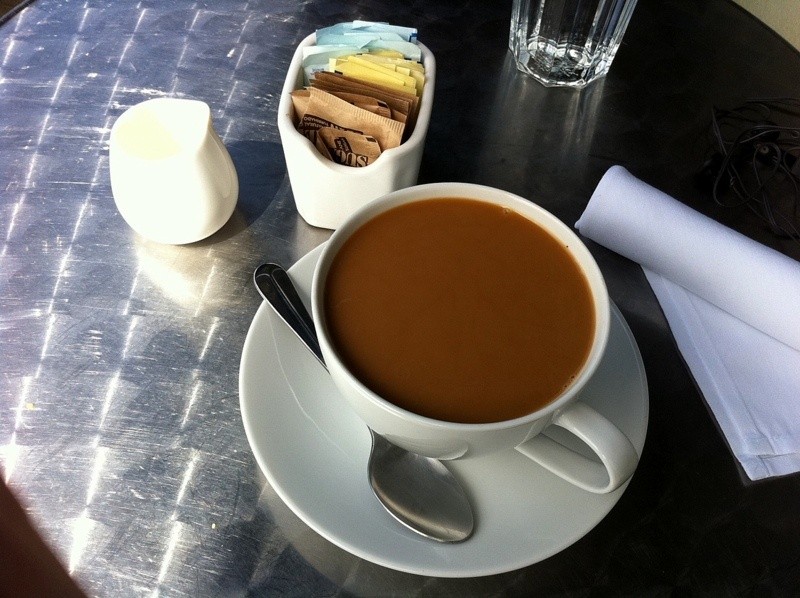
[309,182,638,494]
[508,1,637,88]
[108,98,240,245]
[277,31,436,231]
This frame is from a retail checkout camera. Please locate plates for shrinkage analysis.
[238,239,649,579]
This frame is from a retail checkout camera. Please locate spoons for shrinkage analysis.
[253,262,476,544]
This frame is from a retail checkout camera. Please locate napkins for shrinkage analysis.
[572,165,800,482]
